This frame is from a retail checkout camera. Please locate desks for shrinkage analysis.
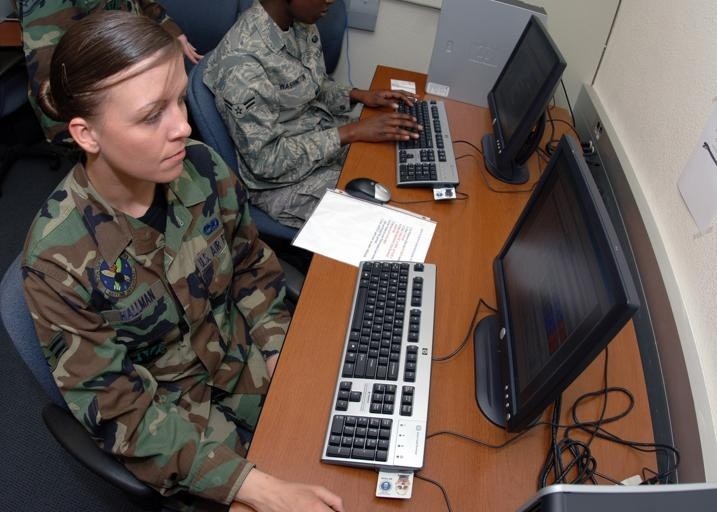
[228,64,664,512]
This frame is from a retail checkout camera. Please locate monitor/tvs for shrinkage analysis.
[472,135,642,432]
[481,14,569,185]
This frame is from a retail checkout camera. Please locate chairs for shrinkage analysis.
[1,254,146,492]
[188,49,293,246]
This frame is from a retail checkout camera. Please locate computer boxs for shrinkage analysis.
[517,483,716,511]
[425,1,549,110]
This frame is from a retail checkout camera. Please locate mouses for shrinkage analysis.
[345,178,392,204]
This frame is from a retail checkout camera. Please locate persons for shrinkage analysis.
[16,1,205,165]
[18,10,343,512]
[395,474,411,498]
[203,0,423,228]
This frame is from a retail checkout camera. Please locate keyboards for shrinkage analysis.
[395,99,459,189]
[319,261,437,474]
